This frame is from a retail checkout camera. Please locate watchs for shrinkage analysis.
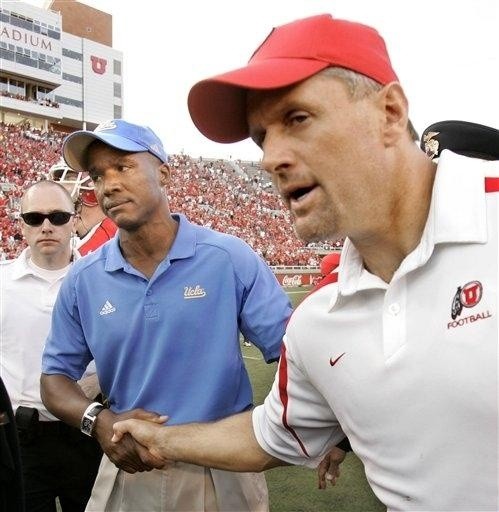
[80,400,105,440]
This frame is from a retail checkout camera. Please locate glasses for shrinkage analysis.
[19,211,77,226]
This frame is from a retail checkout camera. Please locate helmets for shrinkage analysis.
[49,160,100,214]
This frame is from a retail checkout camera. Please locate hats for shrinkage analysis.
[62,119,168,172]
[420,120,499,160]
[187,15,407,144]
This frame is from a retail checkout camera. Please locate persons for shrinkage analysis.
[0,179,108,512]
[1,87,347,271]
[40,115,296,512]
[111,11,499,511]
[317,438,352,491]
